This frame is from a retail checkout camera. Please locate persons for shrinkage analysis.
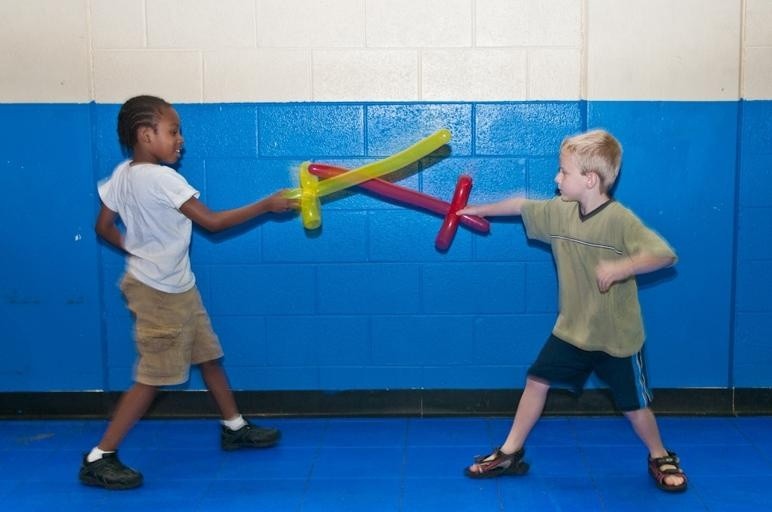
[454,128,689,492]
[80,94,304,490]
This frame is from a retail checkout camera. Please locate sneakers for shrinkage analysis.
[79,448,143,490]
[221,415,280,451]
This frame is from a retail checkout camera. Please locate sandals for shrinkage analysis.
[465,446,528,479]
[648,450,687,493]
